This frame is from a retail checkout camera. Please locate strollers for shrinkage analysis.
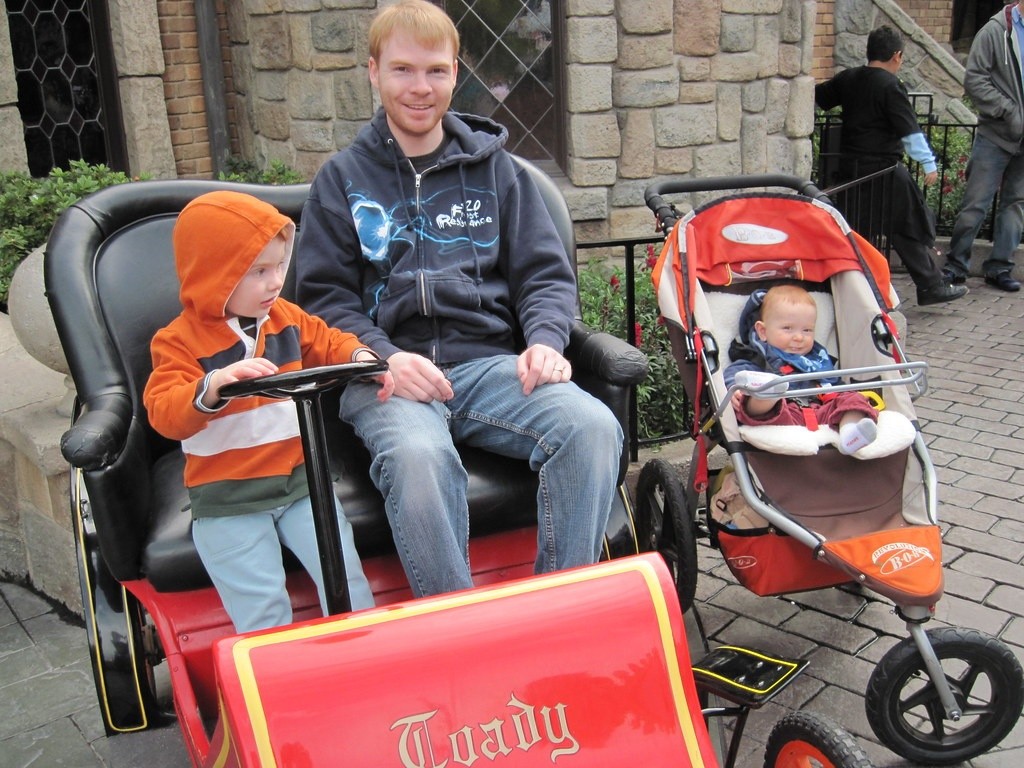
[634,172,1024,768]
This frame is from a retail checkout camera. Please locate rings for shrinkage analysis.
[555,367,564,373]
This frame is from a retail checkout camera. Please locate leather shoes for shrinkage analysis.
[941,260,968,283]
[984,266,1021,293]
[915,284,970,306]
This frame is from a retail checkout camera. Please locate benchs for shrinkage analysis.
[46,178,650,588]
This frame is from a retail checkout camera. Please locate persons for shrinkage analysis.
[142,191,394,634]
[724,284,880,453]
[815,26,971,304]
[937,1,1023,292]
[294,1,626,597]
